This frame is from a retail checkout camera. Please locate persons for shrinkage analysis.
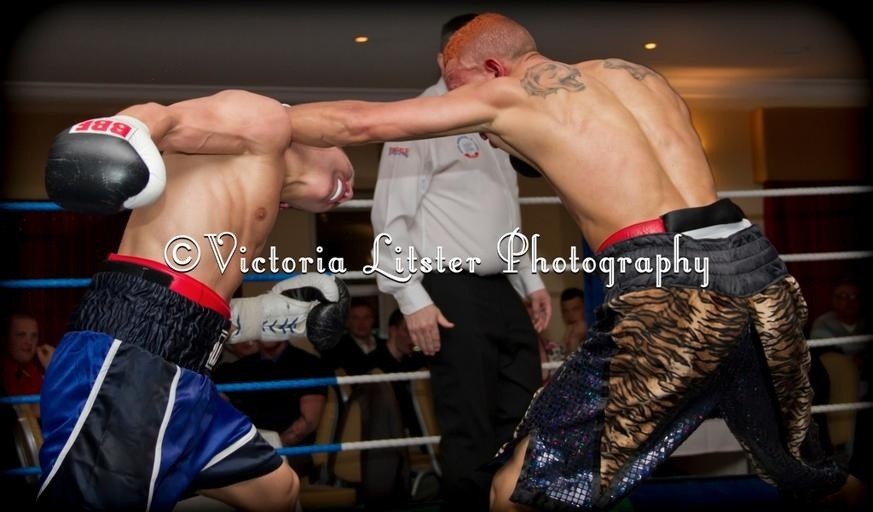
[1,279,872,511]
[366,12,555,510]
[276,10,867,510]
[26,87,358,510]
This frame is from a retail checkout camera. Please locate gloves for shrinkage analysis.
[44,115,166,215]
[227,271,351,350]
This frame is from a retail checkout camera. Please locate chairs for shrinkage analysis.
[290,369,441,511]
[11,402,44,490]
[820,352,858,464]
